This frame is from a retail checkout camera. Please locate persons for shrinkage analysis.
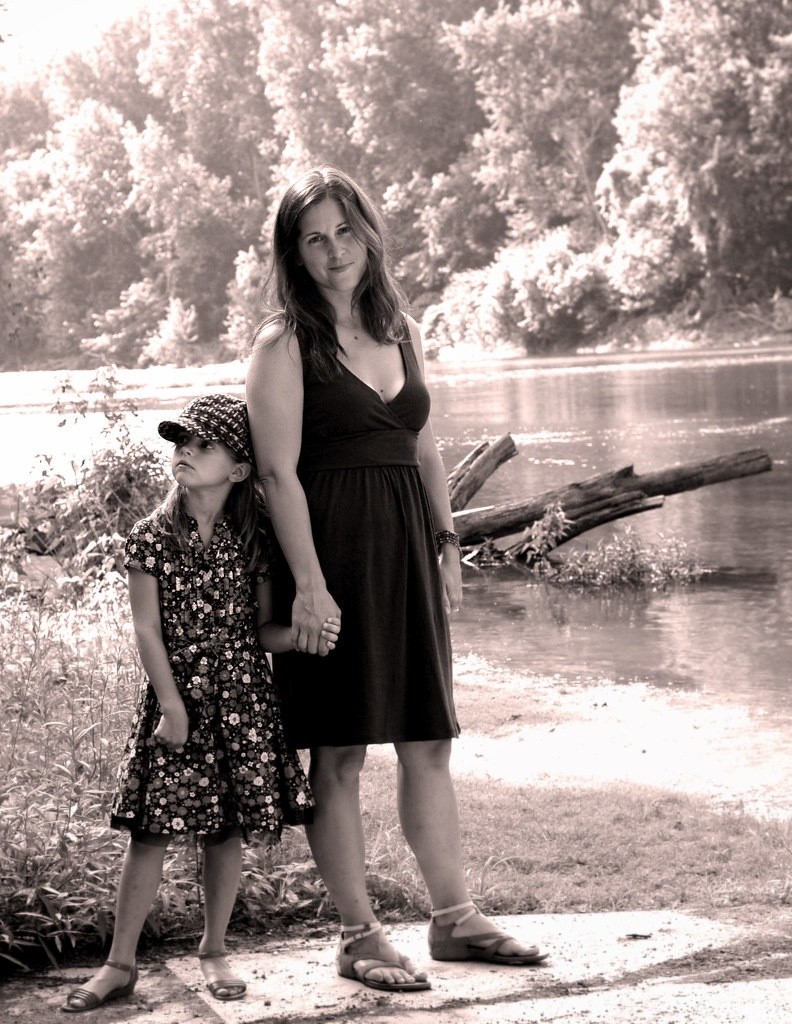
[61,392,341,1012]
[243,167,551,993]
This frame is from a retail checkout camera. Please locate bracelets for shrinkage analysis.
[433,530,464,560]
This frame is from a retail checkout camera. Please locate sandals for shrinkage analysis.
[61,960,138,1012]
[338,921,431,990]
[199,950,247,1000]
[428,901,549,964]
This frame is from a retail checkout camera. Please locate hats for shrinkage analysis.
[159,393,258,476]
